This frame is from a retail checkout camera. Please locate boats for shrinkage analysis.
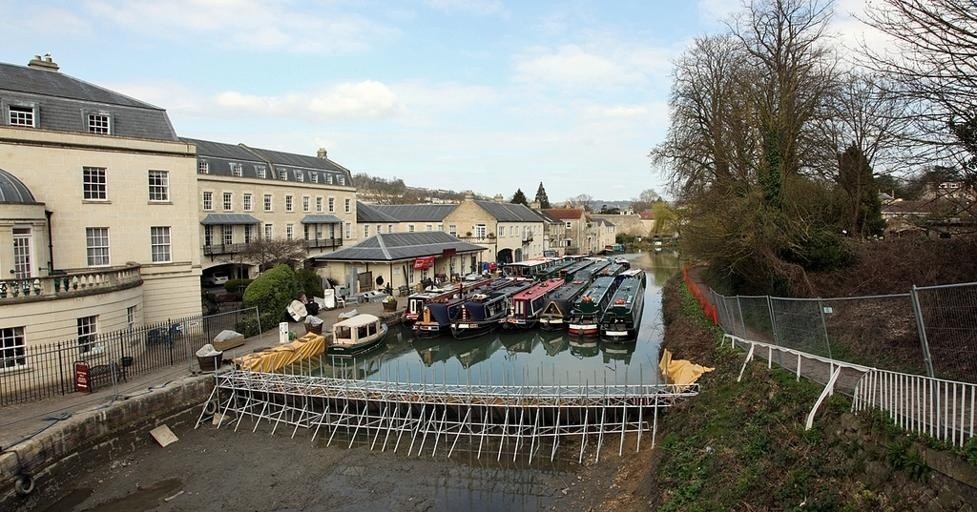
[400,253,647,346]
[326,313,389,360]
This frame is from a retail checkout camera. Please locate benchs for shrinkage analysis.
[88,359,129,392]
